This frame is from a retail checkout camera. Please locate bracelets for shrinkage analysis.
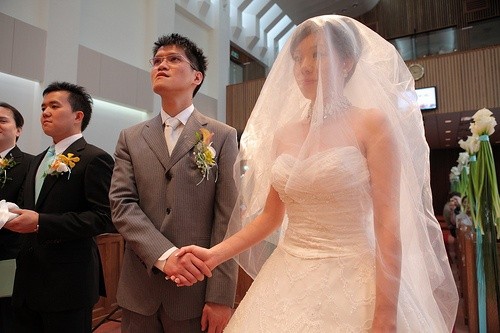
[34,225,39,232]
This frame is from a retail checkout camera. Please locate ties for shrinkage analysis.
[163,118,181,157]
[35,146,55,204]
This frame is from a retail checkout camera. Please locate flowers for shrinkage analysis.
[190,127,219,185]
[0,155,16,183]
[41,153,80,179]
[448,108,500,242]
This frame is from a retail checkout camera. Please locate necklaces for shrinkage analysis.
[308,97,351,124]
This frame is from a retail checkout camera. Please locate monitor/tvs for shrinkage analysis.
[415,86,437,110]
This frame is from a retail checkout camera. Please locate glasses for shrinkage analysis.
[149,55,195,69]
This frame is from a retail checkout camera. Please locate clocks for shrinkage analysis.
[409,64,425,80]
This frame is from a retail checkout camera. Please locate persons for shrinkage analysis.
[443,192,476,237]
[108,33,240,333]
[0,81,116,333]
[165,12,458,333]
